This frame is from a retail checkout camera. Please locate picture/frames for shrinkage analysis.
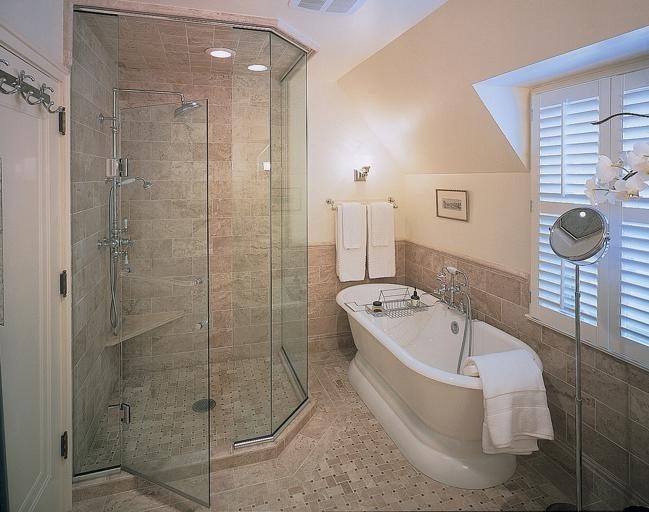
[436,189,468,221]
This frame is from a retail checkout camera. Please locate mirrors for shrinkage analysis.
[549,206,610,262]
[334,203,396,282]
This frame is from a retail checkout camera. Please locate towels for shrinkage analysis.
[463,351,554,456]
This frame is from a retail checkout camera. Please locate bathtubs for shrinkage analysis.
[335,282,544,491]
